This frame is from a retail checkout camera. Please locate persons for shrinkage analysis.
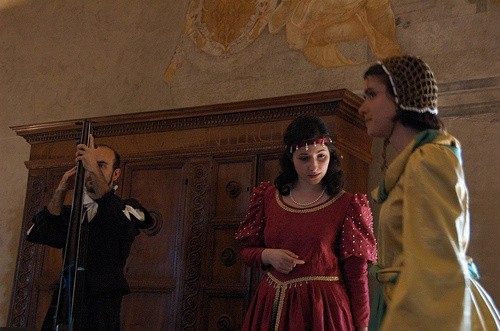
[236,114,378,331]
[358,56,500,331]
[25,134,154,331]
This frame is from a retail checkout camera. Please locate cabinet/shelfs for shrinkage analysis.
[6,89,374,331]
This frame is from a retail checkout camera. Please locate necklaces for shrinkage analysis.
[290,185,326,206]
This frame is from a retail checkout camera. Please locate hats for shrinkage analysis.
[378,54,439,114]
